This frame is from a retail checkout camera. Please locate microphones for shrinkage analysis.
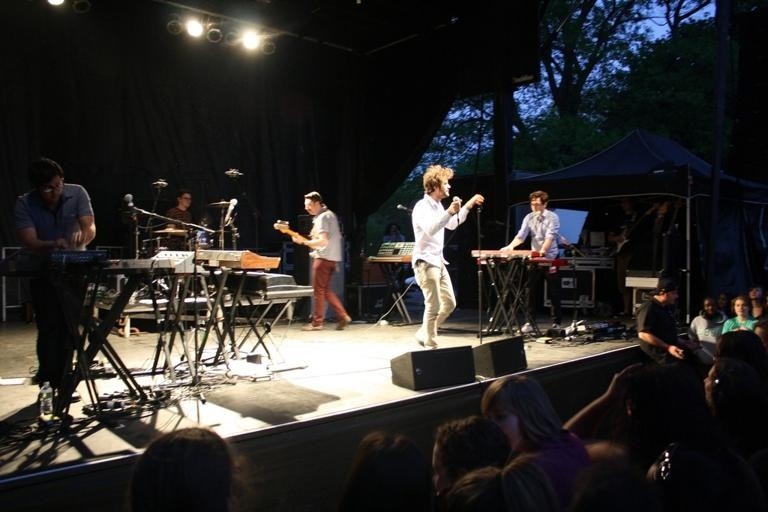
[533,210,541,221]
[397,203,408,211]
[224,168,243,177]
[124,193,134,206]
[453,196,461,223]
[153,177,168,189]
[225,198,238,223]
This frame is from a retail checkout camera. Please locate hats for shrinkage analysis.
[648,277,679,296]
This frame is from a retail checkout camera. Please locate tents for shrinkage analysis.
[503,126,768,327]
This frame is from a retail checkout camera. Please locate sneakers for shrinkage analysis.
[336,315,351,330]
[301,322,323,332]
[552,323,562,329]
[520,322,535,332]
[72,391,82,403]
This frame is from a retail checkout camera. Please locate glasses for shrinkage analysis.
[180,195,192,200]
[43,176,66,193]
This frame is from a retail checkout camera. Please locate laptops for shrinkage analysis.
[590,231,606,247]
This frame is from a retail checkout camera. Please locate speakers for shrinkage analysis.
[391,346,475,391]
[473,336,528,377]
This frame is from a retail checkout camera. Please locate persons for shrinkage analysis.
[753,321,768,349]
[431,416,511,493]
[712,331,765,363]
[341,431,431,512]
[132,426,237,512]
[572,461,652,512]
[499,191,562,335]
[482,376,589,508]
[445,453,559,512]
[748,285,768,319]
[689,298,728,366]
[644,358,760,484]
[167,190,193,250]
[717,293,729,308]
[411,165,485,349]
[721,297,757,333]
[12,159,97,401]
[292,190,352,331]
[561,363,655,467]
[637,274,697,368]
[606,197,669,315]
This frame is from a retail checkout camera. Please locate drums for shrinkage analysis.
[190,230,214,248]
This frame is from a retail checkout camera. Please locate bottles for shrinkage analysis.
[39,382,53,421]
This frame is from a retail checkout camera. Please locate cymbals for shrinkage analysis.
[208,199,230,206]
[153,224,188,233]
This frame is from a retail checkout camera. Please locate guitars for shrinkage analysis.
[612,204,657,252]
[274,220,314,250]
